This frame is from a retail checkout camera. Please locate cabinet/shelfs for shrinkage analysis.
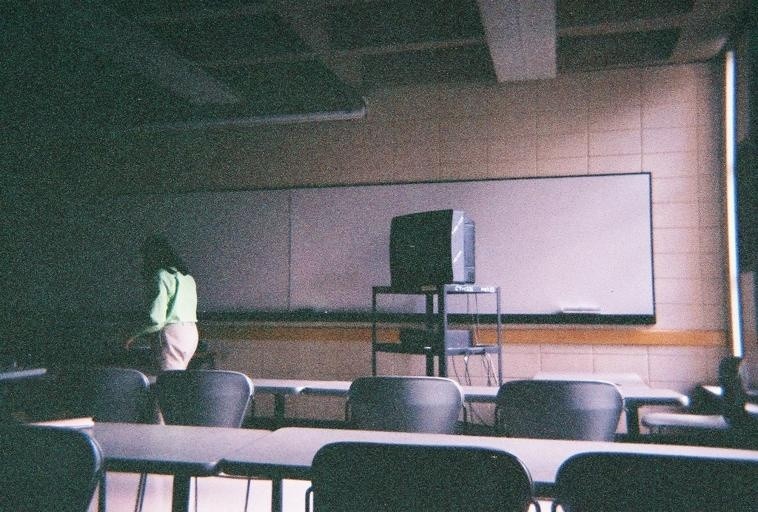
[370,283,502,403]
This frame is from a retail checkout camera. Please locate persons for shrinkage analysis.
[124,234,201,425]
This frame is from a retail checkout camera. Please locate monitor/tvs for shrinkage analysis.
[389,209,475,288]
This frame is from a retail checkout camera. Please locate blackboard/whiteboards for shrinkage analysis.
[0,172,657,324]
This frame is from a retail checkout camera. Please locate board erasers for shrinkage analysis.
[560,306,602,314]
